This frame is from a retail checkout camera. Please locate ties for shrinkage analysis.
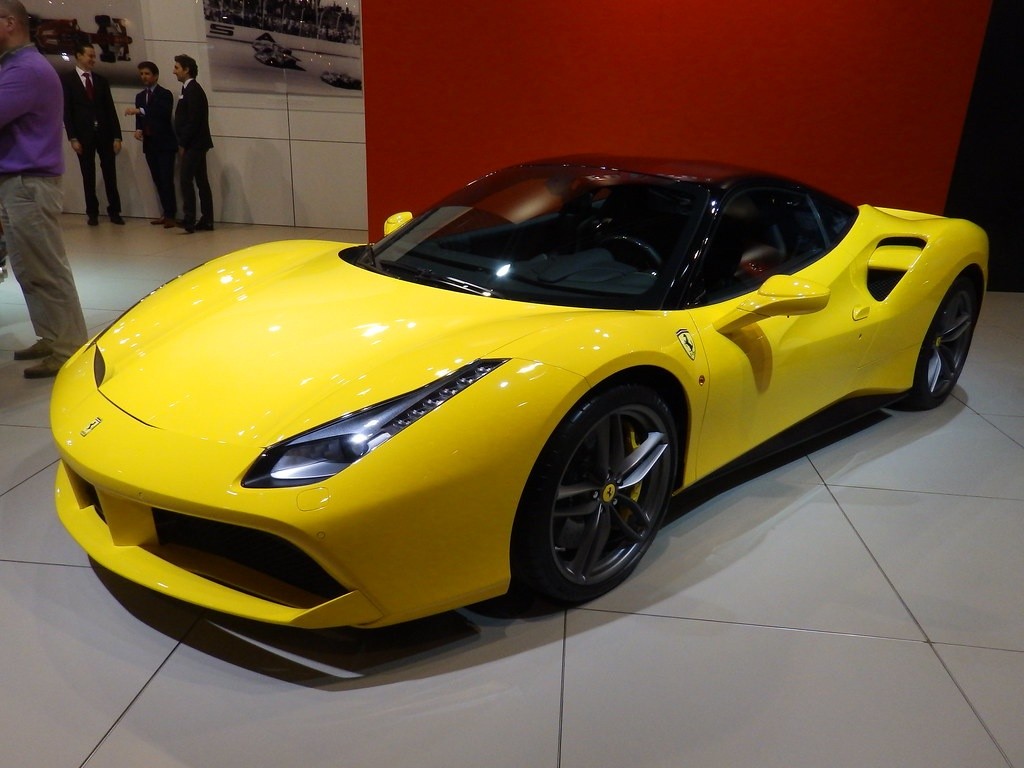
[83,72,94,100]
[181,85,184,95]
[144,89,152,135]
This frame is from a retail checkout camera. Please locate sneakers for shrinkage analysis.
[15,338,54,361]
[23,355,64,378]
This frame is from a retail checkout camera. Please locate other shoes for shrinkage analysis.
[111,216,125,225]
[87,215,98,225]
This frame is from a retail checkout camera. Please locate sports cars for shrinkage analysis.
[48,148,989,631]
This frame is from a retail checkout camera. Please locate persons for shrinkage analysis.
[172,54,216,233]
[0,0,88,378]
[125,61,178,228]
[60,42,126,226]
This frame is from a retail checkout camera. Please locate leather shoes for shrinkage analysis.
[186,221,214,230]
[164,218,174,228]
[177,216,196,224]
[151,215,165,224]
